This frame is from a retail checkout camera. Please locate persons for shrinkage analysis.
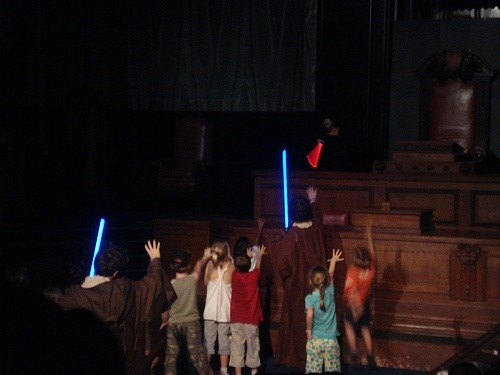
[202,216,268,375]
[0,210,177,375]
[160,247,214,375]
[342,215,376,366]
[274,184,326,370]
[305,248,344,375]
